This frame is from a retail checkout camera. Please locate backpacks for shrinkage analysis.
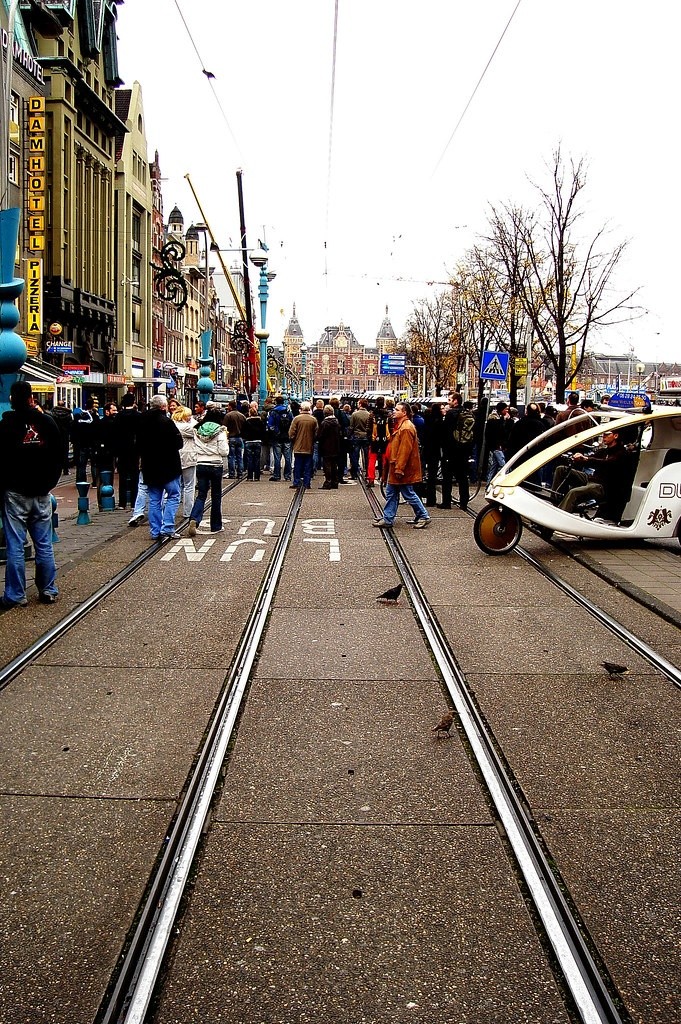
[271,408,290,434]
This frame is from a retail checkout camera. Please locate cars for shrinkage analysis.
[210,391,237,412]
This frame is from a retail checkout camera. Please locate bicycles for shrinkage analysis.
[380,454,480,505]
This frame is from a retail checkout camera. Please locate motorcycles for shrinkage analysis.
[472,393,681,556]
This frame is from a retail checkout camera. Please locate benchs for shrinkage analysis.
[611,447,681,529]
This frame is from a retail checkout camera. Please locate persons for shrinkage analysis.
[288,401,319,489]
[22,390,653,526]
[188,408,229,536]
[372,401,433,528]
[551,428,623,511]
[318,404,342,490]
[0,381,65,607]
[137,395,184,540]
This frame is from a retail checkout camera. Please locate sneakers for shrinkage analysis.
[372,519,393,528]
[413,517,431,528]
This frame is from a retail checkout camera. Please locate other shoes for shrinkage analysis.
[318,484,338,490]
[437,503,451,509]
[41,592,55,602]
[189,520,196,536]
[15,596,27,605]
[460,505,468,510]
[246,478,260,482]
[129,513,144,526]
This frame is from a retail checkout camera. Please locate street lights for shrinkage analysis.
[636,362,646,393]
[250,240,278,416]
[299,344,308,402]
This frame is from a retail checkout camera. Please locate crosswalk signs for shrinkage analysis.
[481,352,509,380]
